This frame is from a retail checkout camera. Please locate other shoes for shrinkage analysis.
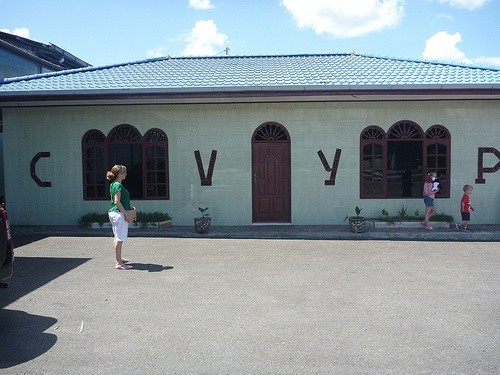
[116,263,133,268]
[463,227,471,231]
[456,223,461,231]
[421,220,426,226]
[121,258,129,262]
[425,224,435,230]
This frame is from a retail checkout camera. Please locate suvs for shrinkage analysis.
[0,205,15,289]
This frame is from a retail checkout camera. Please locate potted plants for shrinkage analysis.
[195,207,211,233]
[343,204,366,234]
[366,208,455,229]
[77,211,172,230]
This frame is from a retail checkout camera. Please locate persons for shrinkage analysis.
[107,165,133,270]
[456,185,473,231]
[420,170,440,230]
